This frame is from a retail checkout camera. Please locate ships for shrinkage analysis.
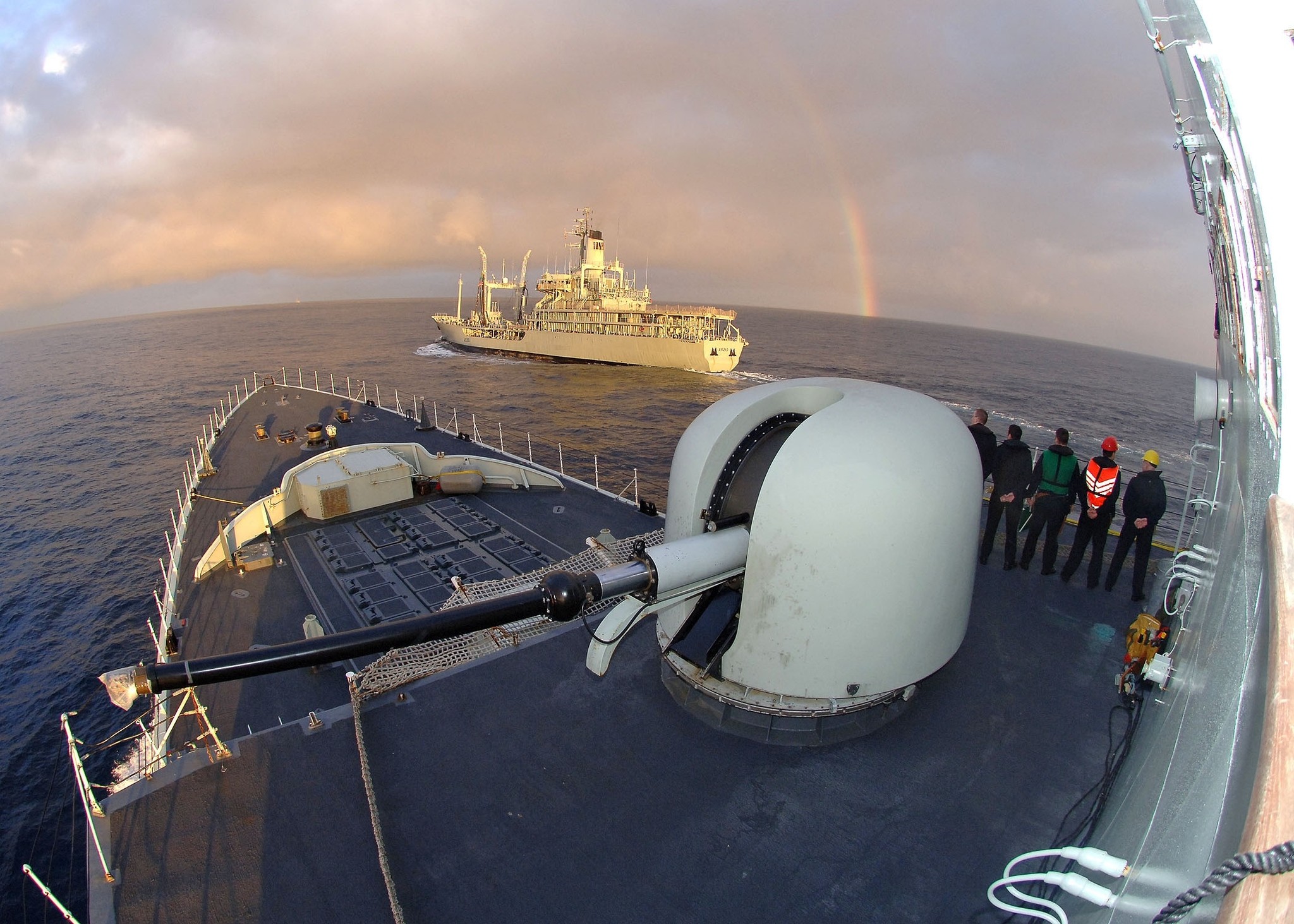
[428,208,749,374]
[14,0,1290,924]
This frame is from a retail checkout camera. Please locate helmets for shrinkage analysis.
[1101,437,1119,452]
[1141,450,1159,466]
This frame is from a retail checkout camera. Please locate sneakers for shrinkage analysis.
[1131,591,1145,602]
[1060,570,1069,583]
[979,555,987,565]
[1087,579,1099,589]
[1041,566,1057,575]
[1105,581,1112,592]
[1018,560,1028,570]
[1004,560,1018,571]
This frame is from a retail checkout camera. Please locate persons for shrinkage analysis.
[1105,450,1167,602]
[966,409,999,495]
[980,425,1034,571]
[1059,437,1122,588]
[1019,428,1081,574]
